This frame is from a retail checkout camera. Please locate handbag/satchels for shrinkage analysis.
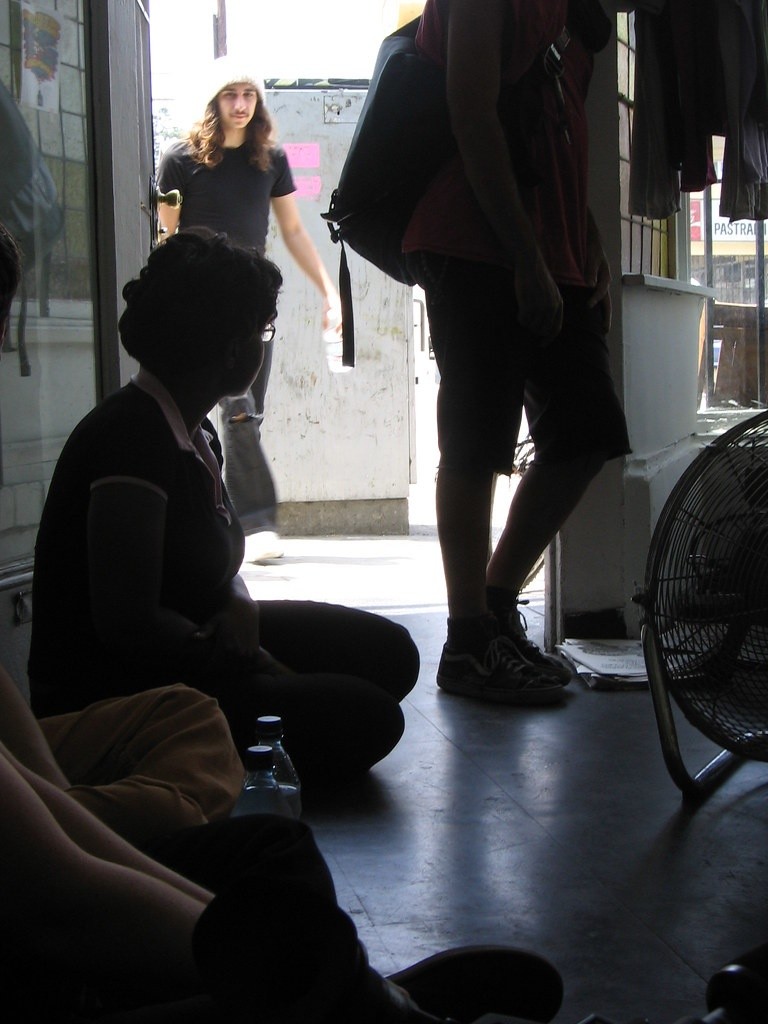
[322,29,514,286]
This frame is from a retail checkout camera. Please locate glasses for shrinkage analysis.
[228,321,276,344]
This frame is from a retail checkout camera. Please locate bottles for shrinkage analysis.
[257,716,302,819]
[324,309,354,373]
[231,746,292,817]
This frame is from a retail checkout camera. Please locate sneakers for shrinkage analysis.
[437,616,563,700]
[487,598,574,686]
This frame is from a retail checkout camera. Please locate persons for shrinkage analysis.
[400,0,628,704]
[157,54,347,567]
[28,227,423,786]
[0,676,567,1024]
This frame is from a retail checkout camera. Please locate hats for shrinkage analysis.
[202,56,266,109]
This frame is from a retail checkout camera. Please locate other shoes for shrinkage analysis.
[384,946,564,1024]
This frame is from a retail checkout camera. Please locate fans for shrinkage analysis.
[629,410,768,795]
